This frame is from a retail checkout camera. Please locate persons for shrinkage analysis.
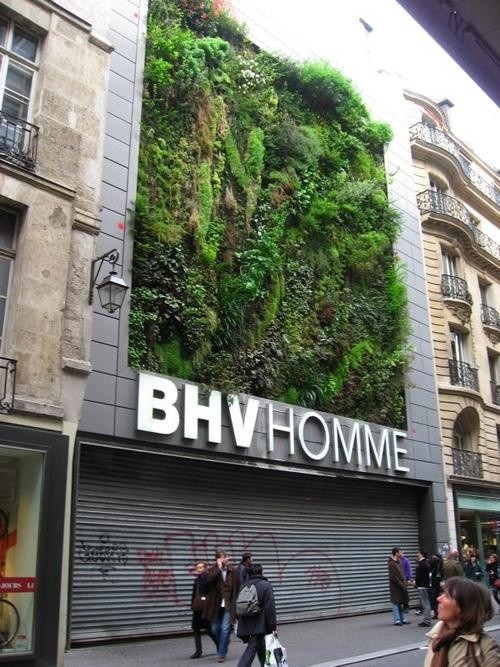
[189,550,277,666]
[388,546,500,627]
[423,577,500,667]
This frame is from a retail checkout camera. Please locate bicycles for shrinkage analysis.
[0,598,20,649]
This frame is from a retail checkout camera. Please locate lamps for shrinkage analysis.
[87,247,131,314]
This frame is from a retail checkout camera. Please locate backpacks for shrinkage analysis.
[236,578,262,616]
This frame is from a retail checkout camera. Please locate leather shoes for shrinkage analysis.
[419,621,430,626]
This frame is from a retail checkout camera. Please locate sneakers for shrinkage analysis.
[415,609,424,615]
[403,618,409,623]
[394,618,401,624]
[215,655,225,661]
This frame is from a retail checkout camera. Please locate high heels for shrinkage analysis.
[190,649,202,659]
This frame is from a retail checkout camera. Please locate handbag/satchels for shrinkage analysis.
[264,632,288,667]
